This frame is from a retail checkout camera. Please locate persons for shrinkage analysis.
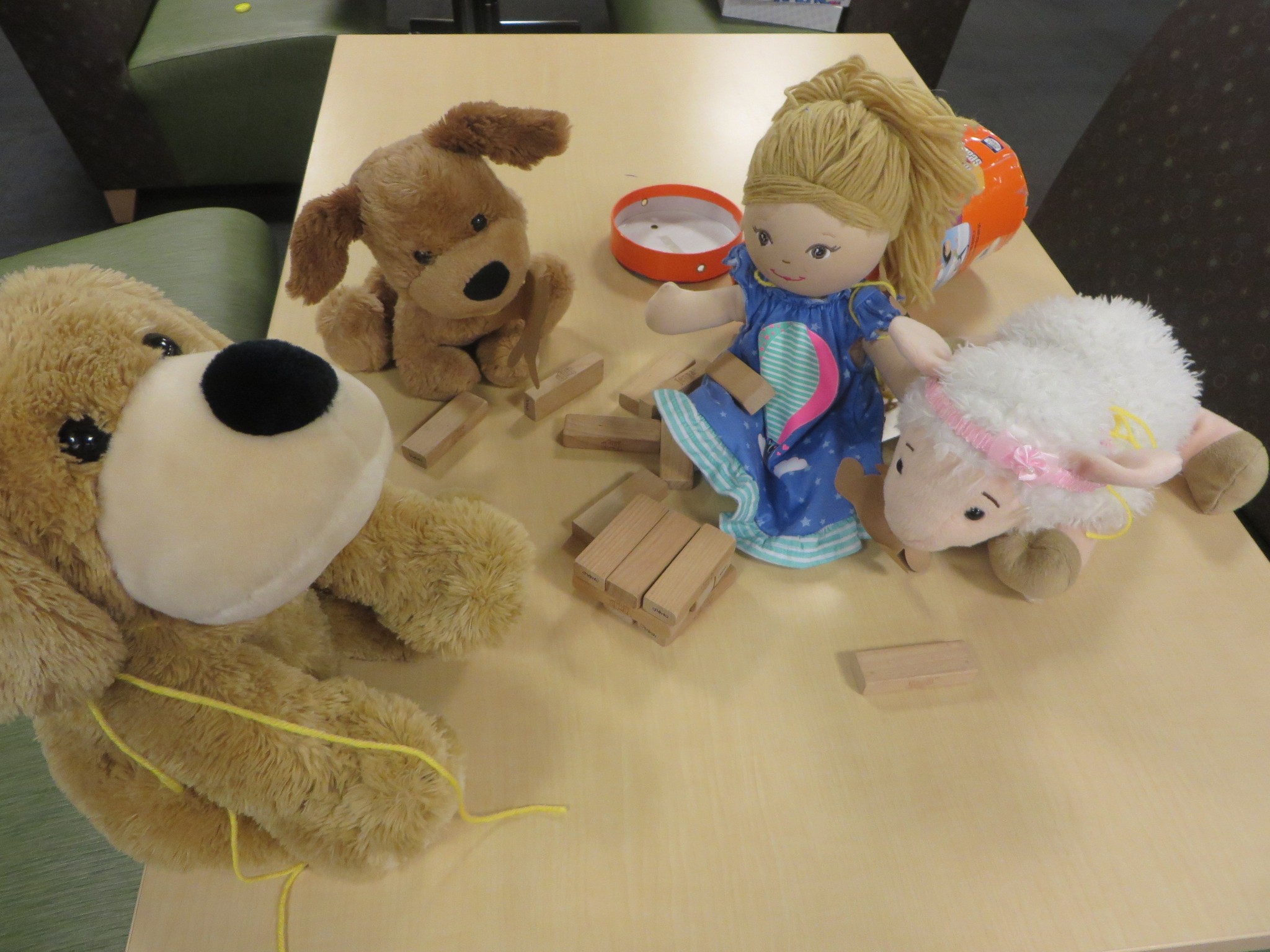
[645,54,985,566]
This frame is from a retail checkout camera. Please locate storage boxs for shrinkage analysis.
[718,0,850,32]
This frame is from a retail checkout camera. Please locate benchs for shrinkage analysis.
[123,35,1270,952]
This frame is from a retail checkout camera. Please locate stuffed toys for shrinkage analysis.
[283,100,576,395]
[0,263,539,880]
[876,291,1269,599]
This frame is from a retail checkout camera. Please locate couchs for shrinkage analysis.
[0,25,275,952]
[0,0,387,190]
